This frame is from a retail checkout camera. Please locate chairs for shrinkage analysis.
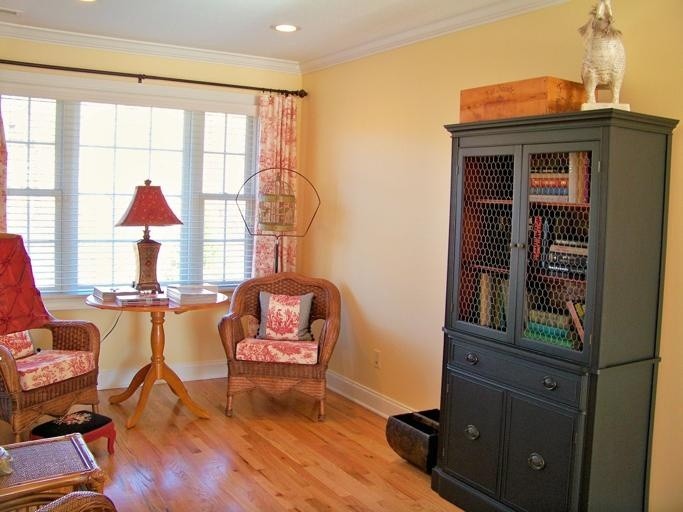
[36,490,117,512]
[0,233,101,443]
[217,271,341,422]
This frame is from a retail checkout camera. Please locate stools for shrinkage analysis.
[30,410,116,455]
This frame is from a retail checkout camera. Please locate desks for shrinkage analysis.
[85,282,229,430]
[0,432,105,503]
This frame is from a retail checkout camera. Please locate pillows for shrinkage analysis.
[255,291,314,341]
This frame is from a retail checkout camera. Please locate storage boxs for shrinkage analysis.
[459,76,598,123]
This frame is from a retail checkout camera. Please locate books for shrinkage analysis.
[472,150,589,352]
[93,282,217,306]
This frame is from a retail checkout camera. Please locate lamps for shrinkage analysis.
[114,179,185,294]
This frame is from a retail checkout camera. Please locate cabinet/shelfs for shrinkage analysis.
[432,108,679,512]
